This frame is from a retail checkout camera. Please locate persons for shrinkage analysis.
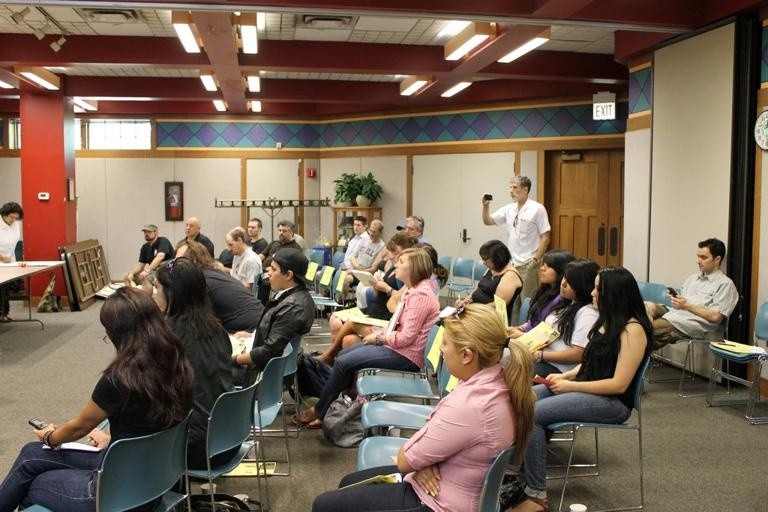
[0,287,198,511]
[502,251,600,384]
[175,241,265,332]
[149,255,244,472]
[125,216,214,286]
[0,200,25,324]
[453,239,523,348]
[643,238,741,353]
[480,176,554,301]
[503,264,655,511]
[219,218,308,290]
[225,248,315,386]
[312,301,535,511]
[293,215,440,426]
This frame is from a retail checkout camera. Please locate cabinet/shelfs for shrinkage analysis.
[331,207,382,249]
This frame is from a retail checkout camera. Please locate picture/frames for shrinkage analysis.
[165,180,184,222]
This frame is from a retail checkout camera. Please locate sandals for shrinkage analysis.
[505,487,553,512]
[289,406,323,428]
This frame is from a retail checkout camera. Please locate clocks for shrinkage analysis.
[755,110,768,152]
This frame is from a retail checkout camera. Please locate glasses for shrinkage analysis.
[512,214,519,228]
[102,335,113,346]
[450,299,476,338]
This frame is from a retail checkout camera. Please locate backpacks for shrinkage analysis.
[284,350,335,403]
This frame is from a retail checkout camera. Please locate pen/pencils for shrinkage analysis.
[87,418,109,442]
[718,343,736,347]
[458,293,460,311]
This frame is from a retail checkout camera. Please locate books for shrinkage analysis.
[40,438,104,453]
[710,337,767,355]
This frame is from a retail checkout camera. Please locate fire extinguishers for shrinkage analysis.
[167,185,181,218]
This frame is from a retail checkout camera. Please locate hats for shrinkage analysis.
[140,223,159,233]
[271,245,309,284]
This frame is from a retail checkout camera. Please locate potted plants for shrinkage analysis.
[354,173,383,207]
[331,174,360,208]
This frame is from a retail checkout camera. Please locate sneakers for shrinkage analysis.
[0,314,13,322]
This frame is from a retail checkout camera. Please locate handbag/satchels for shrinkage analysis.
[322,390,389,450]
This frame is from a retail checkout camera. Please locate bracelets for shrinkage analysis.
[533,257,542,264]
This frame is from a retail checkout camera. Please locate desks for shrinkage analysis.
[58,238,111,310]
[0,259,68,332]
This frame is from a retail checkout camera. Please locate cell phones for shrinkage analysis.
[668,287,677,297]
[485,194,493,200]
[28,417,46,429]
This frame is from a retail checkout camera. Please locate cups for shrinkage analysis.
[201,483,217,494]
[233,493,249,505]
[570,503,588,512]
[246,440,260,460]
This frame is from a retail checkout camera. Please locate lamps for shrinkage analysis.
[11,5,73,55]
[397,21,551,97]
[168,8,263,114]
[0,66,100,114]
